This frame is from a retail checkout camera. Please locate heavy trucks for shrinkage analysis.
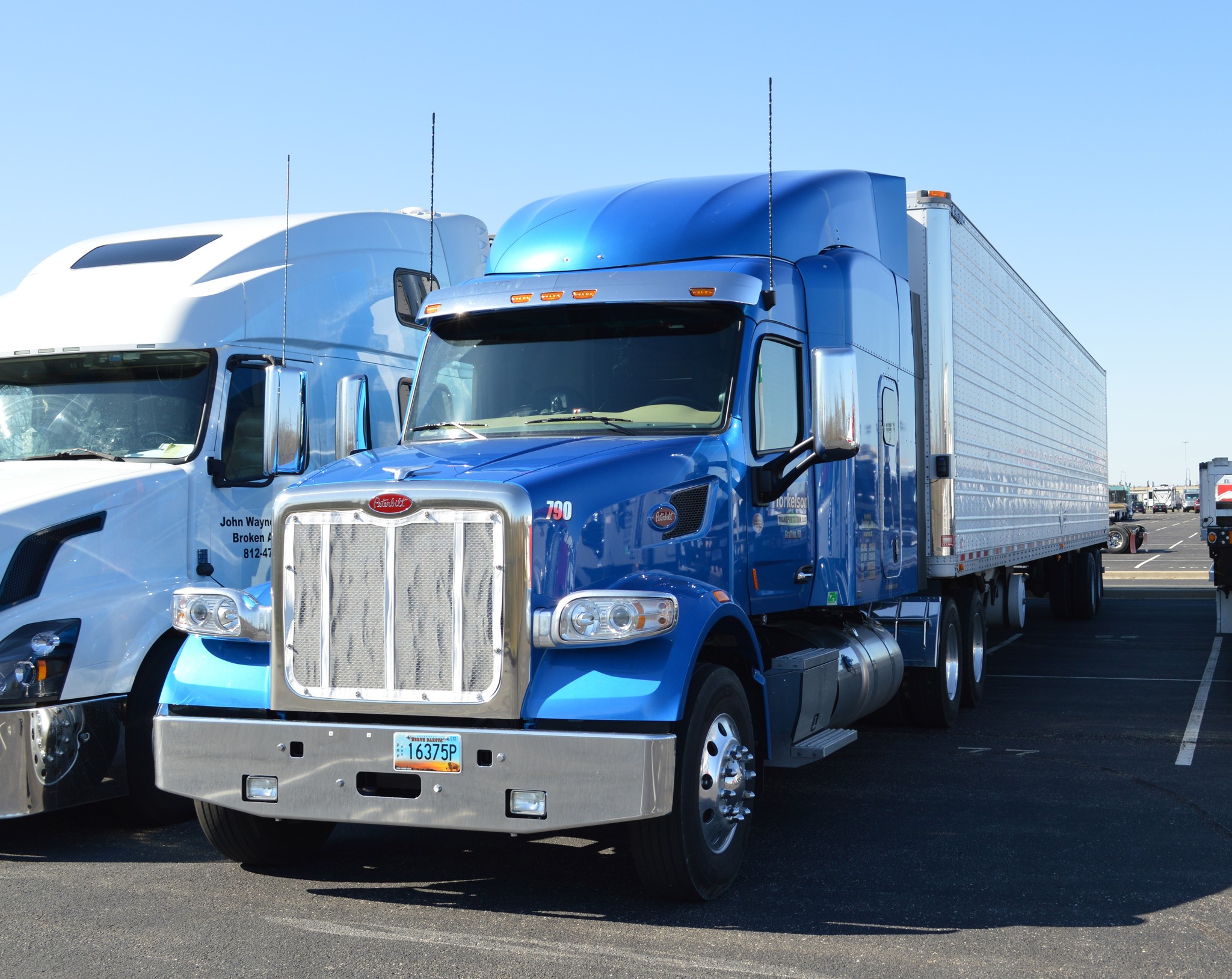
[154,165,1109,900]
[1107,485,1201,553]
[0,209,493,820]
